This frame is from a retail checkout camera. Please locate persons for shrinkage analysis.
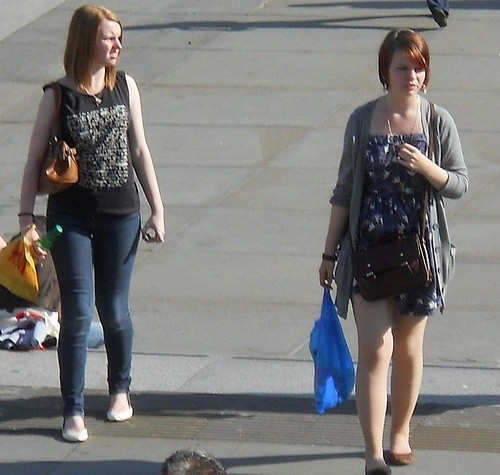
[319,29,469,475]
[19,5,165,441]
[426,0,450,27]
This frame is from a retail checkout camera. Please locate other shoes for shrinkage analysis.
[434,10,449,27]
[107,393,133,421]
[61,416,88,442]
[365,457,391,475]
[389,433,414,464]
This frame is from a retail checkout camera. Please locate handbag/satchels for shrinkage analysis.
[0,214,60,314]
[309,285,356,414]
[351,233,432,301]
[36,80,78,196]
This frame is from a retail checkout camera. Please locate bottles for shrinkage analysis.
[35,225,63,253]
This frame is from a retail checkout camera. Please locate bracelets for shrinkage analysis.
[18,213,35,217]
[322,253,337,261]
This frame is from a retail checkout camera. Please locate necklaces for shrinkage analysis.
[85,85,107,107]
[382,96,419,200]
